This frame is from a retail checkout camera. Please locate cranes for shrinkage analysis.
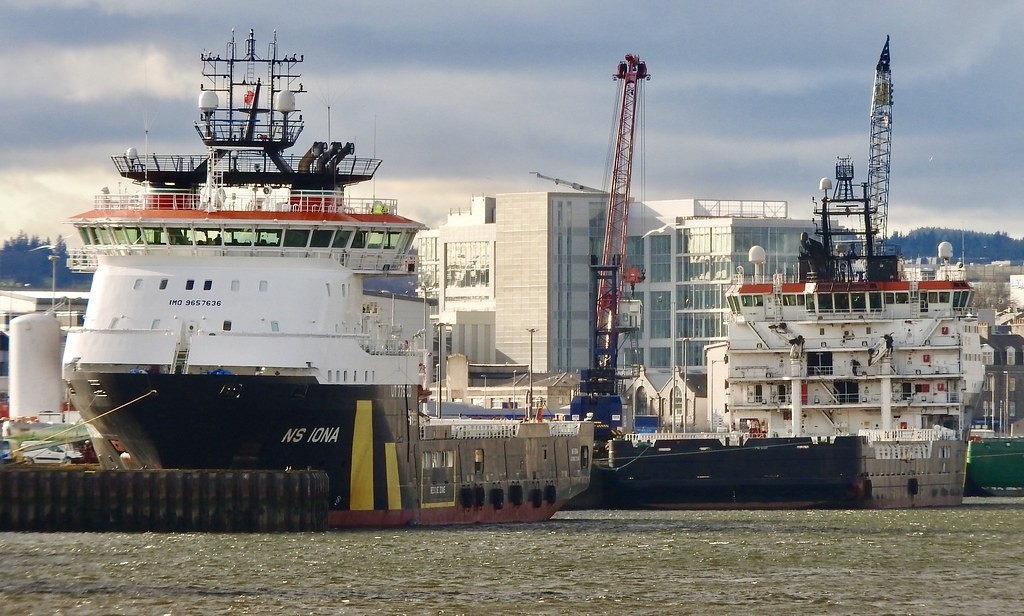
[860,32,896,283]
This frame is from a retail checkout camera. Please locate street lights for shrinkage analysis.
[1003,371,1009,438]
[656,295,691,433]
[480,373,488,410]
[527,327,541,420]
[512,368,518,420]
[681,337,690,434]
[435,364,439,418]
[8,283,31,325]
[381,290,395,327]
[988,372,995,432]
[61,296,83,328]
[48,254,61,318]
[435,322,446,418]
[408,280,427,349]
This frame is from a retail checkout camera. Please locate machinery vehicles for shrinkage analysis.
[562,48,656,461]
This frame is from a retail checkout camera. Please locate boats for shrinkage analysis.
[963,435,1024,499]
[60,24,596,530]
[425,401,872,514]
[701,154,995,509]
